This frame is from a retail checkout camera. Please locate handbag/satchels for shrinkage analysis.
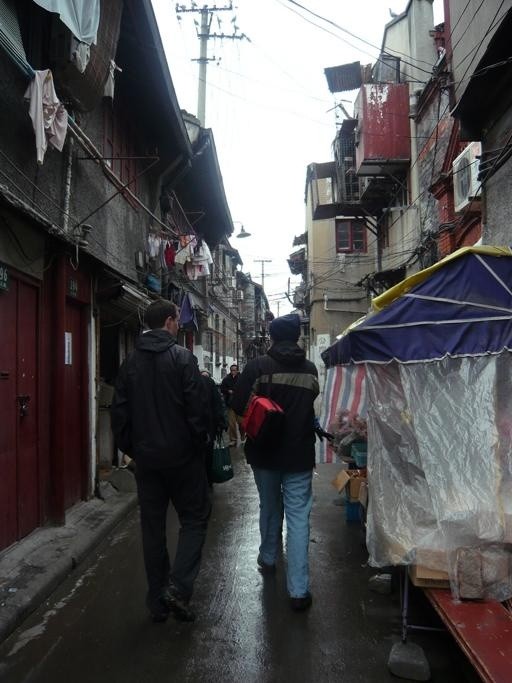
[226,393,242,406]
[209,446,232,484]
[240,393,285,441]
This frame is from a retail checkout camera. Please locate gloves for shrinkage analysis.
[315,427,334,442]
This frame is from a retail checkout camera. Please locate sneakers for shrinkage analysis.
[291,591,312,612]
[147,589,169,623]
[258,554,276,570]
[166,587,196,622]
[229,432,246,447]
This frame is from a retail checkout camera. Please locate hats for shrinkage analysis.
[269,314,301,341]
[200,368,211,375]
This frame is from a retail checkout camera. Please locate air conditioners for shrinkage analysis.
[237,290,244,299]
[227,276,237,288]
[452,141,482,212]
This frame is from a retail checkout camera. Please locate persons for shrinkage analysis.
[227,314,334,614]
[112,298,230,625]
[219,364,246,447]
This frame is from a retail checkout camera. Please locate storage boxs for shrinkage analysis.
[330,442,369,526]
[407,547,452,588]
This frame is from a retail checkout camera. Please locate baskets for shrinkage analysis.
[350,440,367,468]
